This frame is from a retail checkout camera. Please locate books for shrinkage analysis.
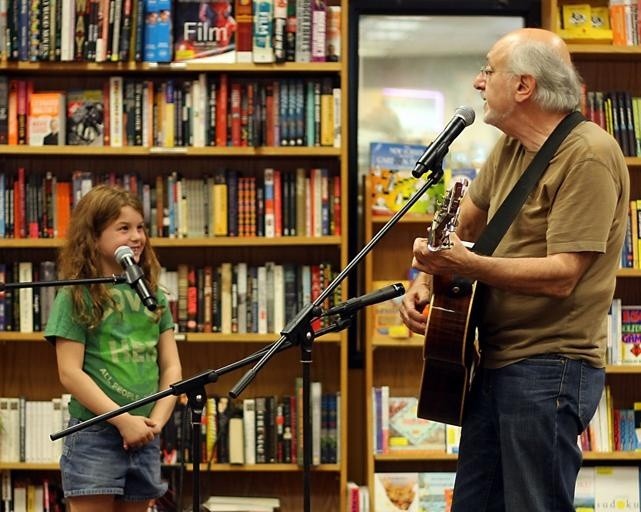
[1,245,72,512]
[368,138,480,512]
[552,1,641,512]
[1,0,341,237]
[155,260,341,512]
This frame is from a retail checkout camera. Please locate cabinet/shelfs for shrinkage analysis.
[542,0,639,462]
[362,172,459,509]
[0,1,350,510]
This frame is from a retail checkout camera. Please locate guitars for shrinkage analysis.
[418,180,478,426]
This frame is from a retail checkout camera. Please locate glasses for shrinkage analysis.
[481,65,515,84]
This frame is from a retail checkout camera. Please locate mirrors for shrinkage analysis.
[352,1,541,371]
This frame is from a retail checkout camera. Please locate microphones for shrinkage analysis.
[113,245,160,312]
[329,283,405,319]
[412,107,475,178]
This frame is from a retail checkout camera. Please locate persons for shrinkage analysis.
[397,28,631,512]
[43,183,183,512]
